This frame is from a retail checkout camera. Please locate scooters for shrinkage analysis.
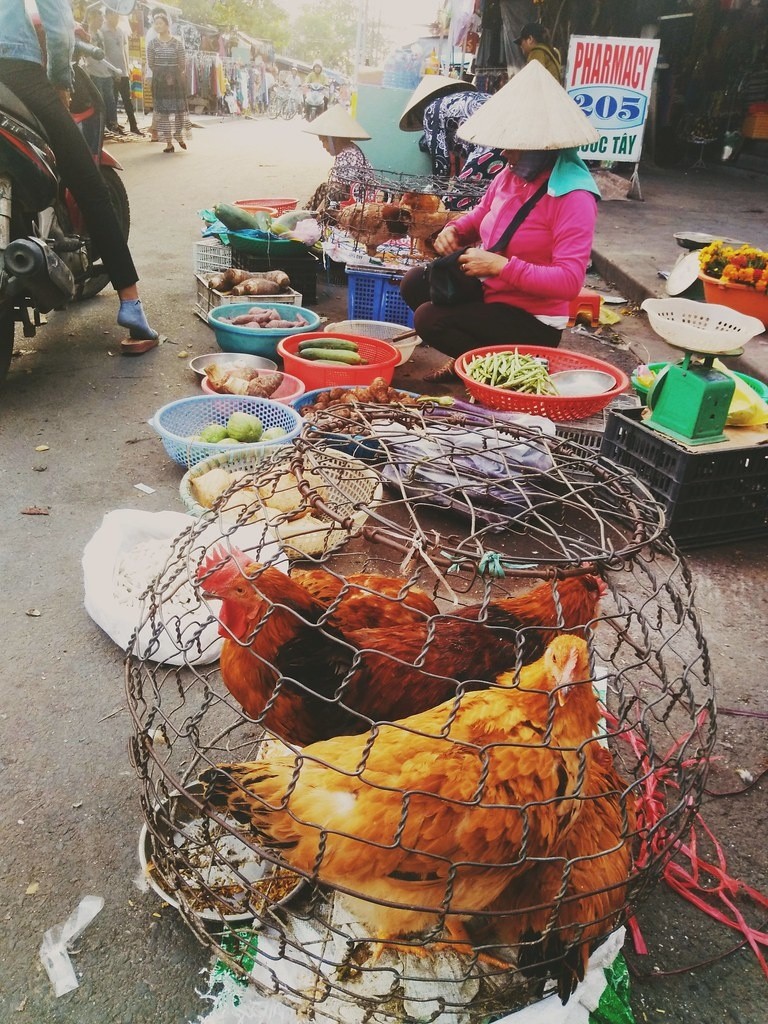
[299,79,336,122]
[0,1,139,392]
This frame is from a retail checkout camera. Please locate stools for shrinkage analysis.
[567,287,600,327]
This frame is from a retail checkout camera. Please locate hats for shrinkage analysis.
[457,59,601,151]
[399,74,477,132]
[513,23,544,46]
[301,103,372,141]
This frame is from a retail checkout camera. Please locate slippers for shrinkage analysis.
[118,328,160,354]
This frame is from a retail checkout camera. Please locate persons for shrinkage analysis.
[99,8,145,136]
[1,0,159,355]
[302,104,374,222]
[398,56,601,384]
[79,8,125,136]
[399,73,507,212]
[284,67,304,114]
[145,8,168,141]
[304,64,329,113]
[147,16,189,153]
[512,24,564,87]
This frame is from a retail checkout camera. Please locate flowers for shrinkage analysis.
[698,239,768,297]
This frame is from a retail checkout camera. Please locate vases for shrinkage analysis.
[698,270,768,330]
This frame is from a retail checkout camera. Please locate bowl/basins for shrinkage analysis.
[199,368,306,405]
[189,352,278,382]
[542,370,616,397]
[207,301,321,363]
[226,230,309,257]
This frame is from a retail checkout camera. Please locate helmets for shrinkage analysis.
[313,59,323,71]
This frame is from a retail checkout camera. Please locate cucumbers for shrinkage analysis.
[295,337,371,366]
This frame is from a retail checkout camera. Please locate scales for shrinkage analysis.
[665,231,751,300]
[640,297,766,446]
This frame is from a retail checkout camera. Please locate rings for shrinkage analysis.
[462,263,465,272]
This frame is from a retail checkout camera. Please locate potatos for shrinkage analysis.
[218,306,310,329]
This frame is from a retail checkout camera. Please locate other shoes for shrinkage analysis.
[131,127,144,135]
[163,145,175,152]
[173,134,187,150]
[115,128,128,135]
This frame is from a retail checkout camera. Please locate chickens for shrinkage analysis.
[198,542,637,948]
[335,188,467,264]
[199,634,600,970]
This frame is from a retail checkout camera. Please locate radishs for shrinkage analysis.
[192,469,328,543]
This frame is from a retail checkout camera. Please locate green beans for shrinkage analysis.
[463,346,562,398]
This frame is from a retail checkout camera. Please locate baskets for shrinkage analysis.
[147,395,305,469]
[201,368,306,415]
[178,441,384,556]
[640,298,767,353]
[235,205,279,220]
[234,198,300,217]
[454,345,630,421]
[324,319,423,367]
[277,331,402,395]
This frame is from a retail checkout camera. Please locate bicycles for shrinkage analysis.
[267,81,301,122]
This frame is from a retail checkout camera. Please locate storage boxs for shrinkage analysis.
[468,393,768,552]
[325,256,429,346]
[192,238,321,327]
